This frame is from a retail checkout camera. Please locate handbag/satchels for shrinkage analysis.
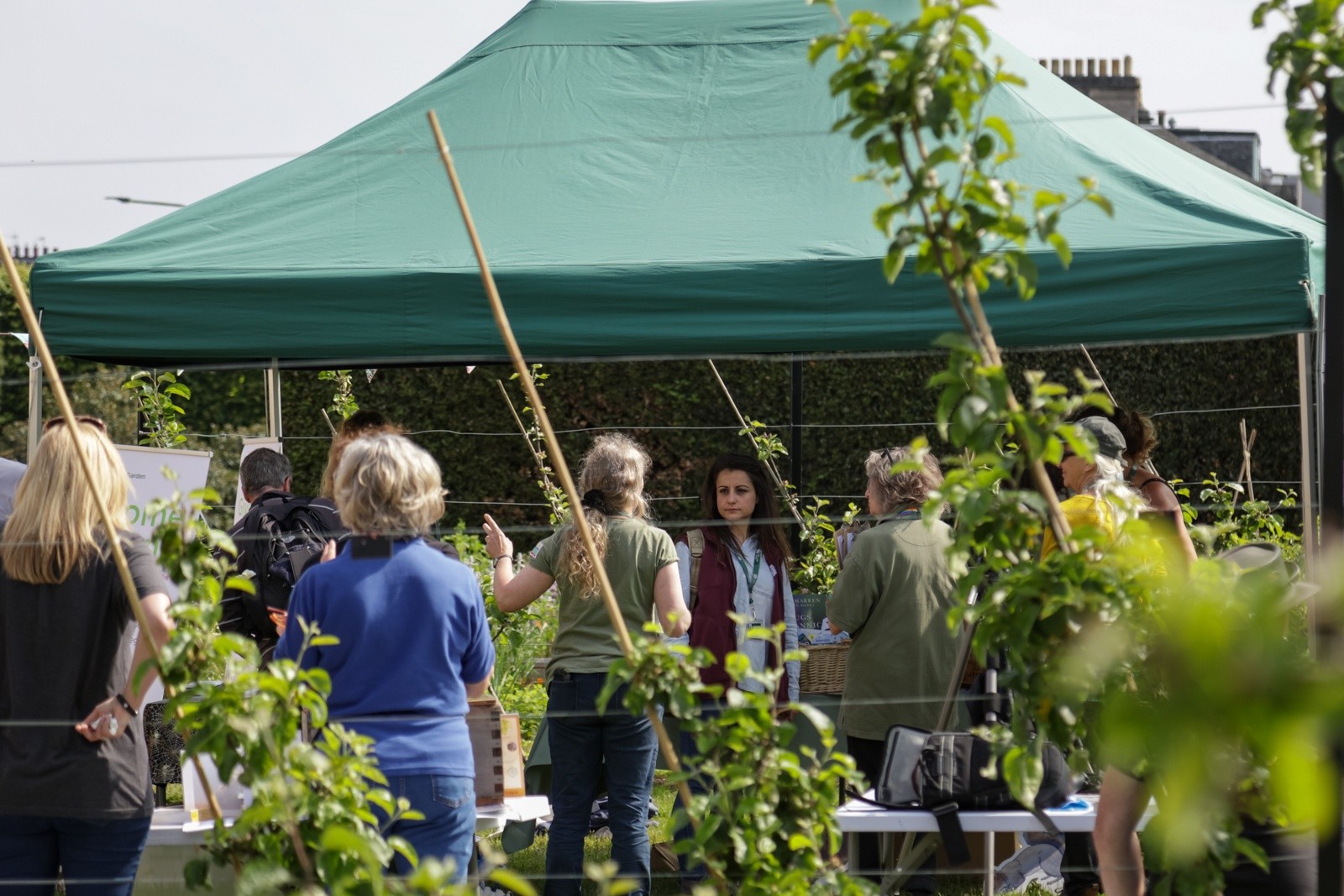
[875,723,1074,814]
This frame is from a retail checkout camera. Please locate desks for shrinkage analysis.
[834,788,1163,896]
[141,807,507,896]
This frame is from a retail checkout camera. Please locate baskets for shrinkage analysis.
[800,639,856,696]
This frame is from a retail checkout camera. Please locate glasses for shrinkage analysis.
[1061,450,1077,464]
[881,449,893,468]
[1276,563,1300,598]
[42,415,106,437]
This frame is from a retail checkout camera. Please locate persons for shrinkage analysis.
[483,434,690,896]
[274,433,495,884]
[207,412,458,667]
[1040,418,1196,896]
[660,454,800,896]
[828,452,977,895]
[0,422,174,896]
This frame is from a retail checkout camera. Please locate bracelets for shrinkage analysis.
[116,692,138,716]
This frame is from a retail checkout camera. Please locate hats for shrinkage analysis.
[1068,416,1127,460]
[1207,538,1319,626]
[1065,402,1128,445]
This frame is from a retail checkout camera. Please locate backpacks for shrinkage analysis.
[241,497,337,642]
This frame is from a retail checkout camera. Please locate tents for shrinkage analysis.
[29,0,1325,568]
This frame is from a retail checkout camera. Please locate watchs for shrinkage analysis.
[493,554,514,569]
